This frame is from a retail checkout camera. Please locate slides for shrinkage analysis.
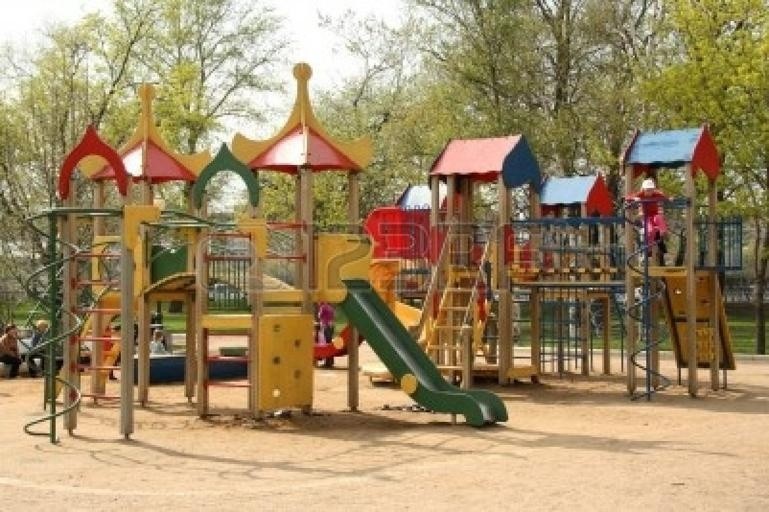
[338,279,508,426]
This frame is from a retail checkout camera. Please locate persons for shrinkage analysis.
[150,330,167,355]
[26,321,49,378]
[318,300,335,369]
[626,180,669,266]
[0,325,21,379]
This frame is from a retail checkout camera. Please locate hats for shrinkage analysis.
[642,179,655,189]
